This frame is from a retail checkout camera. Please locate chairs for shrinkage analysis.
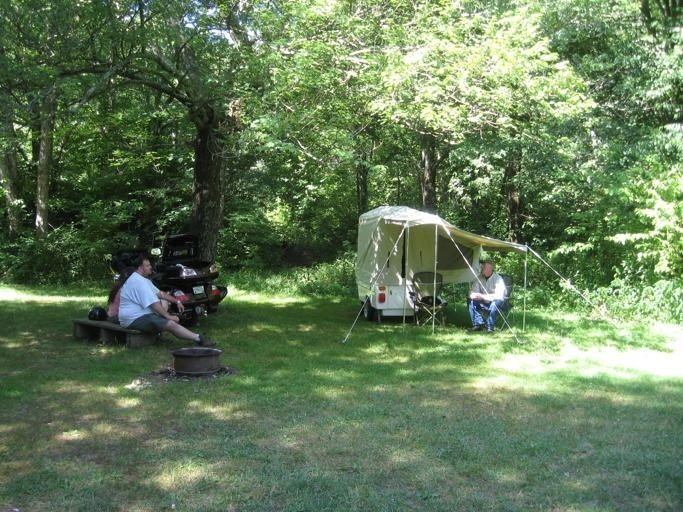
[467,275,515,331]
[409,272,448,327]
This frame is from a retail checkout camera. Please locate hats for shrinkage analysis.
[478,260,493,264]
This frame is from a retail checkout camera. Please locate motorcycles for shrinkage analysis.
[102,231,227,327]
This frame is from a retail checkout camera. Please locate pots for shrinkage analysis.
[169,347,222,375]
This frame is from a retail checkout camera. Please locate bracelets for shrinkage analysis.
[174,300,182,305]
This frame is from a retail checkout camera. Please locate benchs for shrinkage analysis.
[72,318,156,348]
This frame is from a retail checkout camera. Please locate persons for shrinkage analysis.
[117,253,215,347]
[466,258,508,334]
[105,266,135,325]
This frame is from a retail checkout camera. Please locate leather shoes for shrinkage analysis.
[465,325,481,333]
[487,326,494,334]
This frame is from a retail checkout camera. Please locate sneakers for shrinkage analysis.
[195,334,215,347]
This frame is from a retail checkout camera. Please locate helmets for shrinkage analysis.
[89,307,106,320]
